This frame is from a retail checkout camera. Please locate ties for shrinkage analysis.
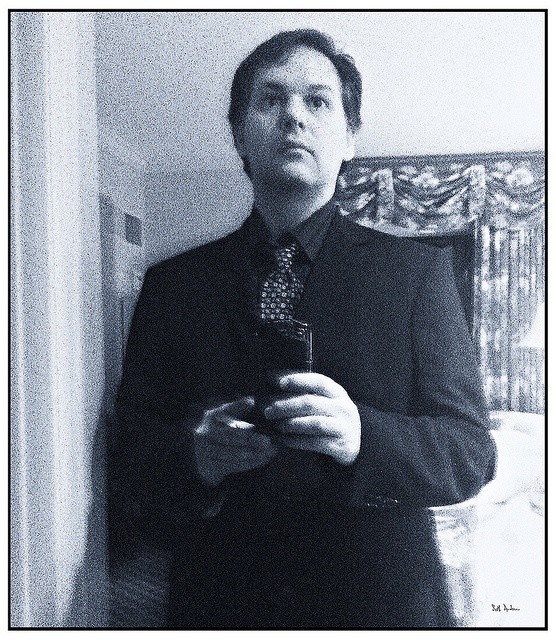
[260,241,304,324]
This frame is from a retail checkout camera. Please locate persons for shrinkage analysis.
[109,28,498,627]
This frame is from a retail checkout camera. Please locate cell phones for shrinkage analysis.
[254,320,313,433]
